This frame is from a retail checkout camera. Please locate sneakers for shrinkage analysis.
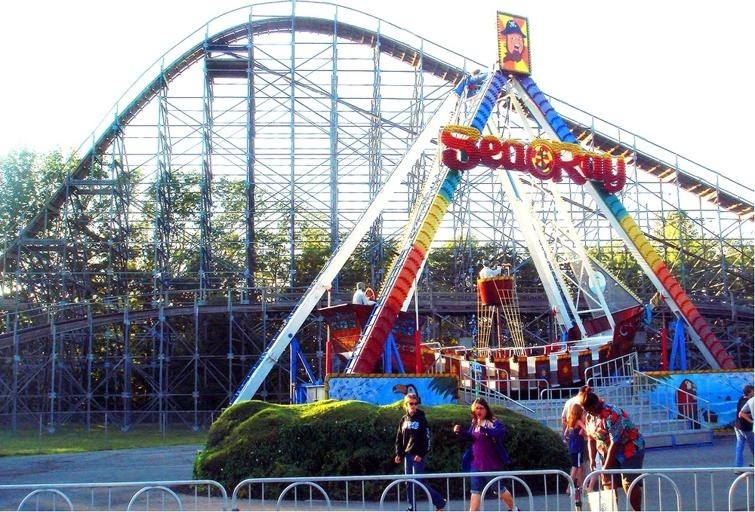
[734,471,744,474]
[436,499,447,511]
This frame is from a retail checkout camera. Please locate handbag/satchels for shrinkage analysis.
[587,489,618,512]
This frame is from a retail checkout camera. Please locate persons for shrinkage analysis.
[733,385,754,474]
[738,395,754,431]
[478,260,512,277]
[394,393,448,511]
[560,385,646,511]
[452,398,520,511]
[352,282,371,305]
[365,287,376,302]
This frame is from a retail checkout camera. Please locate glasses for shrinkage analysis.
[410,402,419,406]
